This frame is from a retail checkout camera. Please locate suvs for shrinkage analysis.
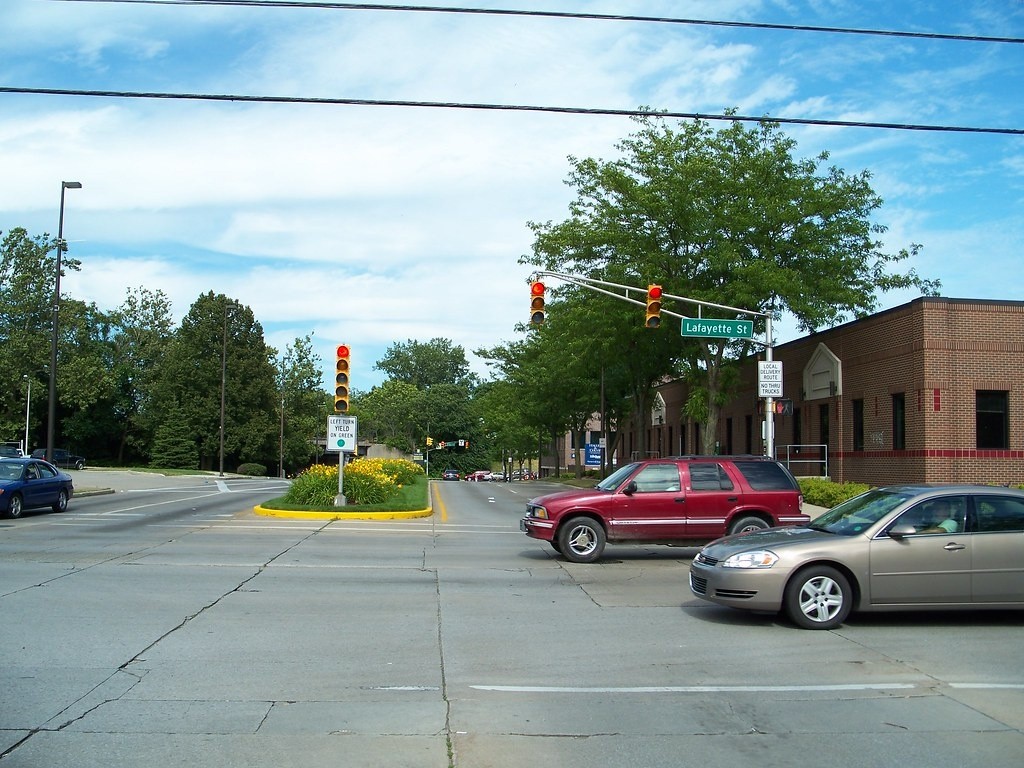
[30,448,86,470]
[519,453,810,564]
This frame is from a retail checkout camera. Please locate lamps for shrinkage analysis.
[799,388,806,401]
[830,381,837,396]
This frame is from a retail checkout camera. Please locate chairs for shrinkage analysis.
[921,506,933,529]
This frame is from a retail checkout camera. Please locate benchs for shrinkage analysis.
[989,510,1024,531]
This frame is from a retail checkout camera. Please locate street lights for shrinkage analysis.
[280,356,293,478]
[22,375,32,455]
[45,181,85,465]
[220,304,238,476]
[315,403,327,465]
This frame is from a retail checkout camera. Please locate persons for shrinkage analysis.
[915,501,958,534]
[638,473,676,492]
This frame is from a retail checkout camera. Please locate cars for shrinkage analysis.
[463,468,538,482]
[443,469,461,481]
[684,483,1024,630]
[0,445,32,458]
[0,458,73,519]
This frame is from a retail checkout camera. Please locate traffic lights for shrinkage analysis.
[465,442,469,449]
[644,284,663,328]
[530,281,546,325]
[427,437,433,446]
[441,441,445,449]
[775,399,794,416]
[334,343,350,413]
